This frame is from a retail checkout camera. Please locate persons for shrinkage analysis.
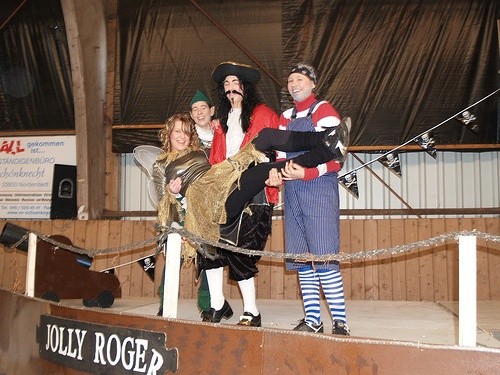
[157,89,215,323]
[202,62,280,326]
[151,111,352,259]
[263,58,350,336]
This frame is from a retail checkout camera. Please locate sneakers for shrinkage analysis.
[322,116,352,163]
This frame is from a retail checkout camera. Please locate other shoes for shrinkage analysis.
[332,320,350,335]
[293,318,323,333]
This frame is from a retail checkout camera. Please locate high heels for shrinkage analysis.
[202,300,233,323]
[237,312,261,327]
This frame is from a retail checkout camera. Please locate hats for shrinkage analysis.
[190,89,210,105]
[288,62,317,85]
[211,61,260,85]
